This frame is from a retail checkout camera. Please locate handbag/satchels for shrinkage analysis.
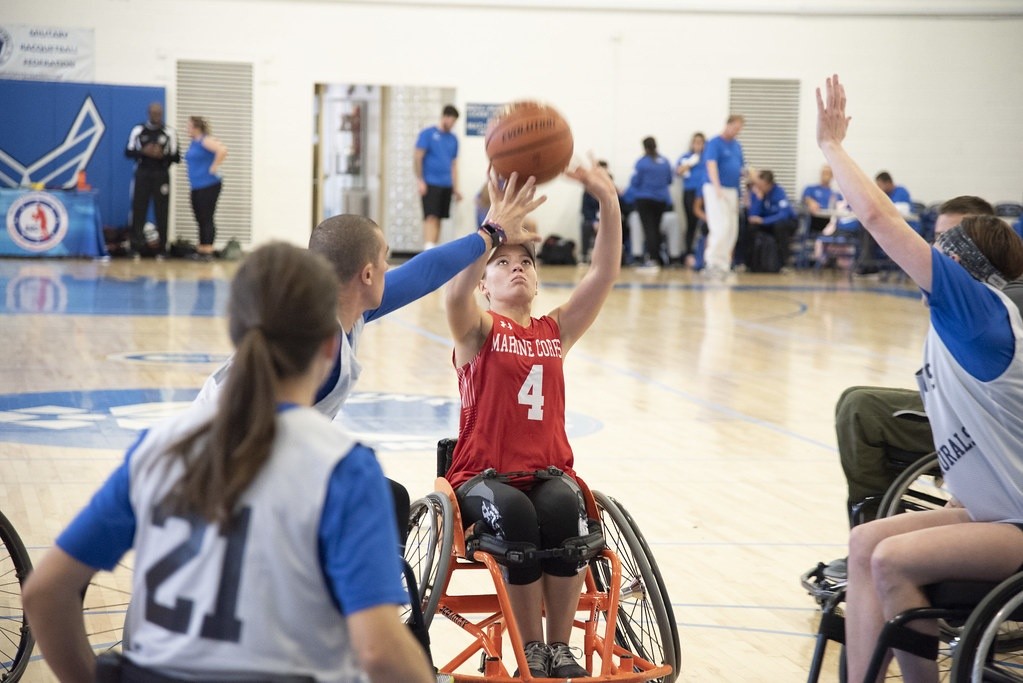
[220,239,243,261]
[535,235,577,265]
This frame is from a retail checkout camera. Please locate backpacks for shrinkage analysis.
[745,231,781,274]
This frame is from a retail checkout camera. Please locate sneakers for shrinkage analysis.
[548,642,591,678]
[512,641,554,678]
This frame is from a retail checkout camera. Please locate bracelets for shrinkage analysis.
[481,220,507,246]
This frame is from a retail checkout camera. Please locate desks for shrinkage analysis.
[0,188,111,260]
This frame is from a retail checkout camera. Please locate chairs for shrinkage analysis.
[788,201,1023,285]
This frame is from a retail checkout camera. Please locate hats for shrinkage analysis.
[479,226,535,302]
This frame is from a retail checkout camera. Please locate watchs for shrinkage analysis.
[477,225,499,249]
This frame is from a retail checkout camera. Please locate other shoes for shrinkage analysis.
[822,556,848,579]
[854,264,879,280]
[632,259,660,272]
[187,251,214,263]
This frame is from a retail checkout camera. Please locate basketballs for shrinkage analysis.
[484,99,575,187]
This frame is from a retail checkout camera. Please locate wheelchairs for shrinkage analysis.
[799,409,1023,683]
[398,439,683,683]
[0,512,316,683]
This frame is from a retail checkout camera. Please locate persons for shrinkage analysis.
[185,117,225,258]
[796,166,859,270]
[701,114,746,275]
[446,150,623,677]
[815,73,1023,682]
[197,171,547,424]
[477,172,536,243]
[676,133,708,272]
[579,136,675,269]
[414,105,463,250]
[22,240,441,683]
[821,195,994,581]
[734,171,800,273]
[849,172,911,278]
[124,101,181,258]
